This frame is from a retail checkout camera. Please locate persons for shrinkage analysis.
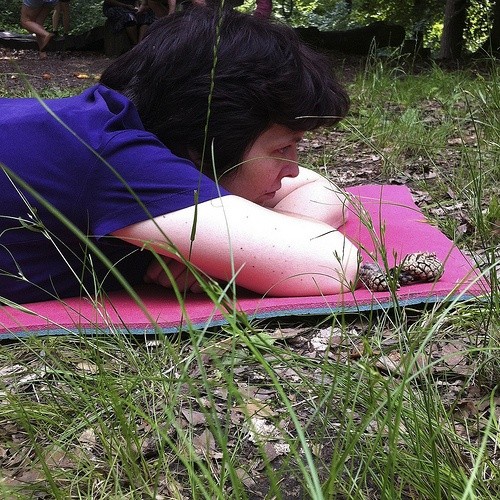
[19,0,243,50]
[0,7,366,295]
